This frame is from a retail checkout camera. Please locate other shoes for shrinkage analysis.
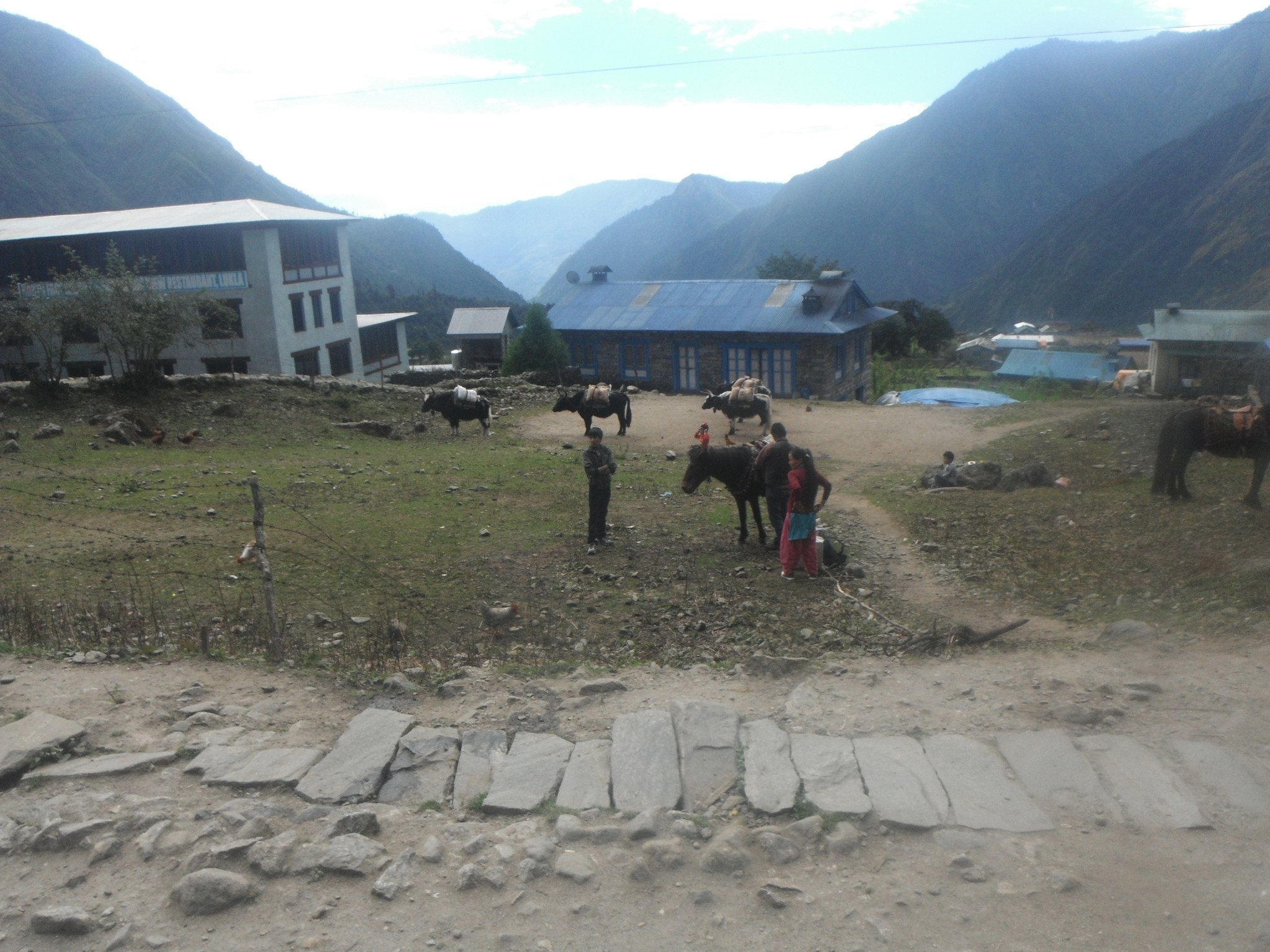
[764,542,780,550]
[808,574,818,579]
[587,543,599,553]
[597,537,614,545]
[781,570,796,580]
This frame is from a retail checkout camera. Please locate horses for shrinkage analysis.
[1151,404,1270,510]
[680,433,769,547]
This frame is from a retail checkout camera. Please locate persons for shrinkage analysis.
[934,451,958,487]
[779,448,832,580]
[582,427,617,555]
[694,422,712,483]
[754,422,804,549]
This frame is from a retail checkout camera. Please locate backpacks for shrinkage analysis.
[819,530,848,567]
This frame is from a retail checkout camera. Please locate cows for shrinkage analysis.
[701,379,772,436]
[553,387,633,436]
[418,385,493,437]
